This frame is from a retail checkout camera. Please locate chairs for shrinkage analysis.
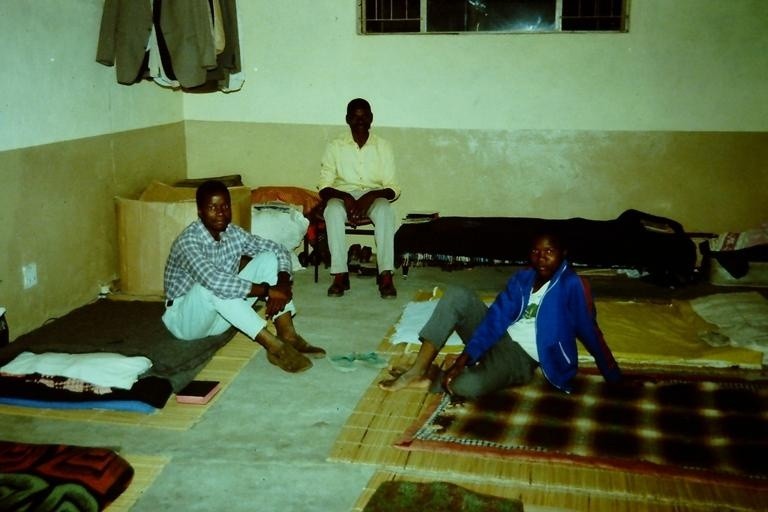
[312,204,382,285]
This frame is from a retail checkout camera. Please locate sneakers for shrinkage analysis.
[275,333,327,359]
[326,276,350,297]
[298,252,330,267]
[348,244,372,264]
[377,271,398,300]
[268,341,313,375]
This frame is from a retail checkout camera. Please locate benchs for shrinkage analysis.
[390,211,696,284]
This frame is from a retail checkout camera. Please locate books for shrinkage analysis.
[176,380,221,406]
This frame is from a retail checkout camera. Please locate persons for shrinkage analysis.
[161,180,326,372]
[377,233,648,400]
[318,99,401,299]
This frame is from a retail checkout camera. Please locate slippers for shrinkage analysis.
[328,350,359,373]
[353,351,389,369]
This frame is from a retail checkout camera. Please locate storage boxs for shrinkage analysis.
[112,178,252,296]
